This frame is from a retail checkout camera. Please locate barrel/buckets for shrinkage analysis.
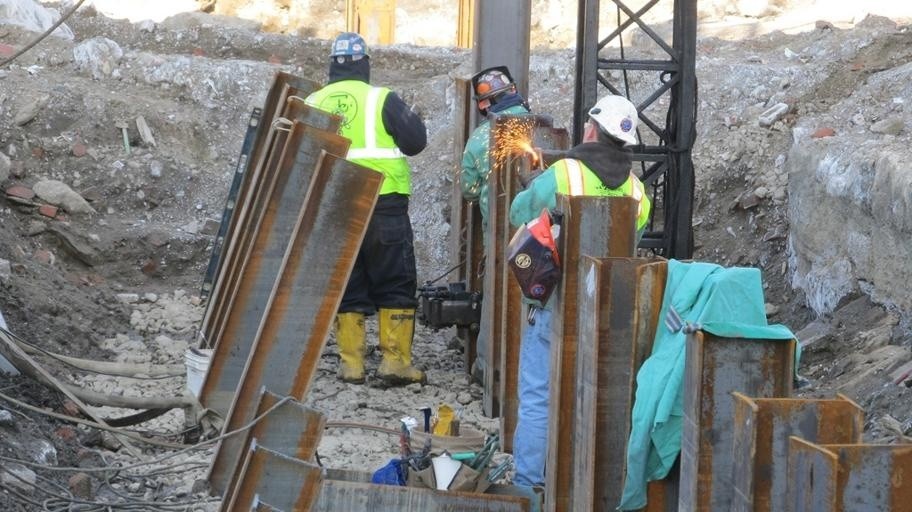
[184,331,215,399]
[410,425,499,469]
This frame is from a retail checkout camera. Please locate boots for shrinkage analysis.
[375,306,427,386]
[332,311,367,384]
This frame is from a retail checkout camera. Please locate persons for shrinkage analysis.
[303,30,429,386]
[507,94,651,512]
[458,66,530,385]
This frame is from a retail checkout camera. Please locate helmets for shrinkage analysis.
[328,32,370,59]
[588,95,638,146]
[525,207,560,265]
[476,70,517,109]
